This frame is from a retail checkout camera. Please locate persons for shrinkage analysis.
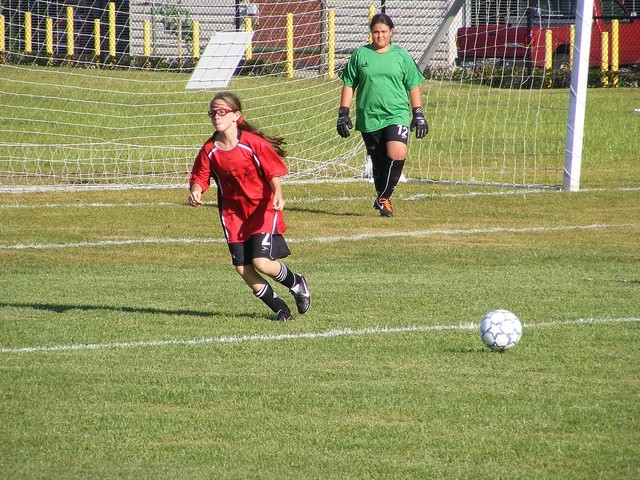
[336,15,428,219]
[186,92,310,322]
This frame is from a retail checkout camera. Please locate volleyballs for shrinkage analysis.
[480,308,522,352]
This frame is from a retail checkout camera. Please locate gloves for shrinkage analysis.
[409,106,429,139]
[336,106,353,138]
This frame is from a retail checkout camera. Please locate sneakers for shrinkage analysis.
[275,304,291,321]
[288,273,312,315]
[373,198,394,219]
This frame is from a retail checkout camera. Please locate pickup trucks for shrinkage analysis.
[456,0,640,78]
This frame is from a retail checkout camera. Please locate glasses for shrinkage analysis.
[207,109,239,117]
